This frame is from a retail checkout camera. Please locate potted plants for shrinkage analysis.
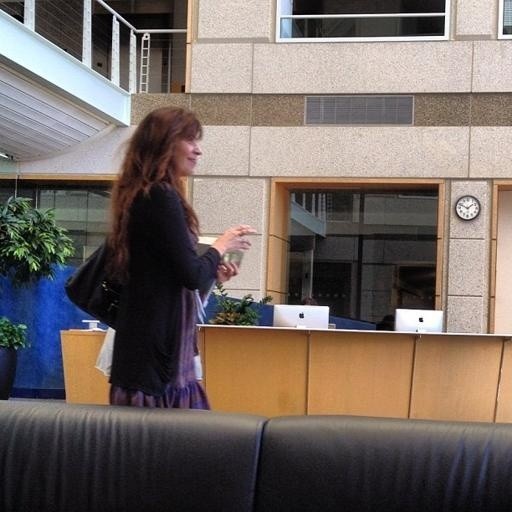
[0,315,34,400]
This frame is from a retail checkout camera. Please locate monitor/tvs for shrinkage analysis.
[394,306,445,333]
[272,304,330,329]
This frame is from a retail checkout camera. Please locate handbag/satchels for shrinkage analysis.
[65,233,123,332]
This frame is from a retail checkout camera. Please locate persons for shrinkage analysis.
[108,105,260,411]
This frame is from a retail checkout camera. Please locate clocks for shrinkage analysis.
[455,195,481,222]
